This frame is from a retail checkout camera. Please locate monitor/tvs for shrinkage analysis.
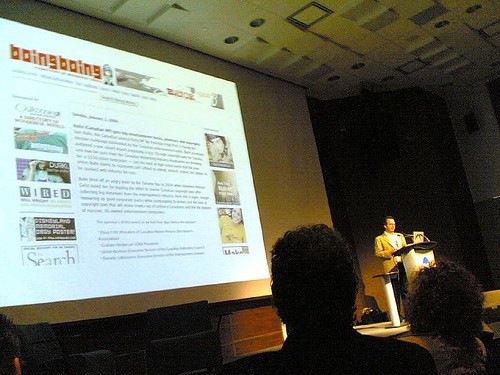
[413,232,424,244]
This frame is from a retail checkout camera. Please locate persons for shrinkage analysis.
[391,254,487,375]
[219,208,246,243]
[1,313,30,374]
[211,221,440,375]
[206,133,232,164]
[374,215,408,273]
[22,159,48,182]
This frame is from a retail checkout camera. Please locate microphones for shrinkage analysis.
[396,233,430,242]
